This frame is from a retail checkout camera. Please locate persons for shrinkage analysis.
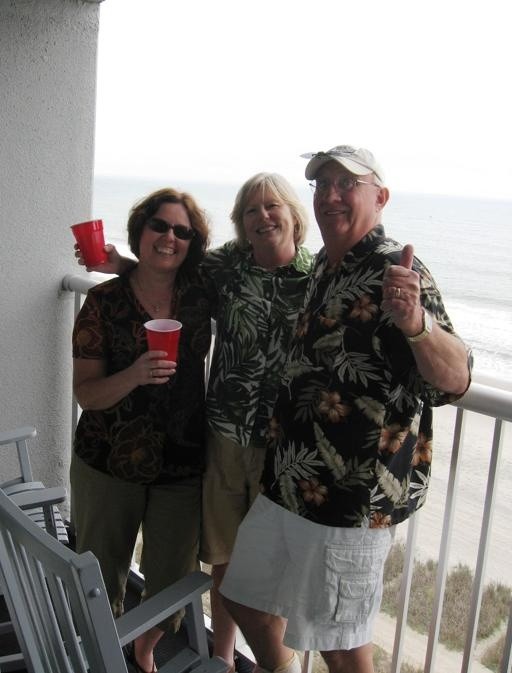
[66,190,210,673]
[68,171,317,671]
[219,143,475,671]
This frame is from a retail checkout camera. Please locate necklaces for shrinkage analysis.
[133,278,176,315]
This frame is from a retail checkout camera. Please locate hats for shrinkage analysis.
[305,144,387,185]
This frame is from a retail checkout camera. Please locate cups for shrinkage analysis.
[144,319,183,378]
[70,218,108,266]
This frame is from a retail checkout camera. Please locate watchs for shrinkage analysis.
[401,308,432,344]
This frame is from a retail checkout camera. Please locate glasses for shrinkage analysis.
[147,217,198,240]
[309,176,383,194]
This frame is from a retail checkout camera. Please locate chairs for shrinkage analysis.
[0,489,231,673]
[0,428,69,672]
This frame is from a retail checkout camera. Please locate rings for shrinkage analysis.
[394,286,402,298]
[148,370,155,378]
